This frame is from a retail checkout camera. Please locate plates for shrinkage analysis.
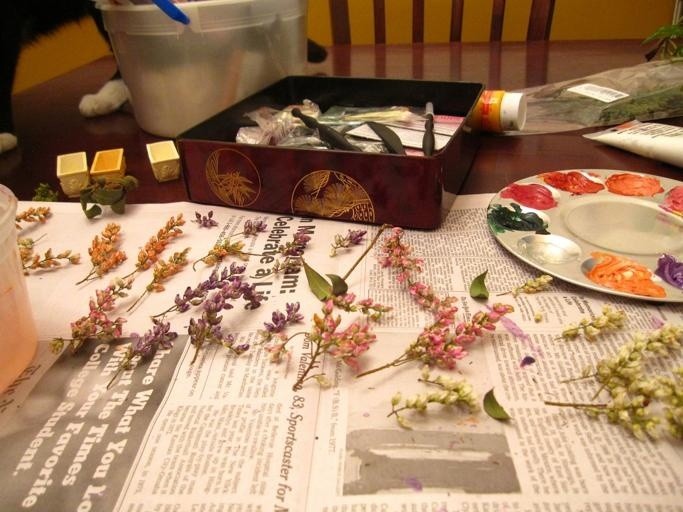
[486,167,682,302]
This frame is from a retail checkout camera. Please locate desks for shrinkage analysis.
[0,38,683,512]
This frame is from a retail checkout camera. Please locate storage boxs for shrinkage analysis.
[175,75,486,231]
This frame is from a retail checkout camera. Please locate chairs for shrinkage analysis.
[329,0,556,45]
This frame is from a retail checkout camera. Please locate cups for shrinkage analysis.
[1,185,38,397]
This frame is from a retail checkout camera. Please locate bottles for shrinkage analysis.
[463,88,530,134]
